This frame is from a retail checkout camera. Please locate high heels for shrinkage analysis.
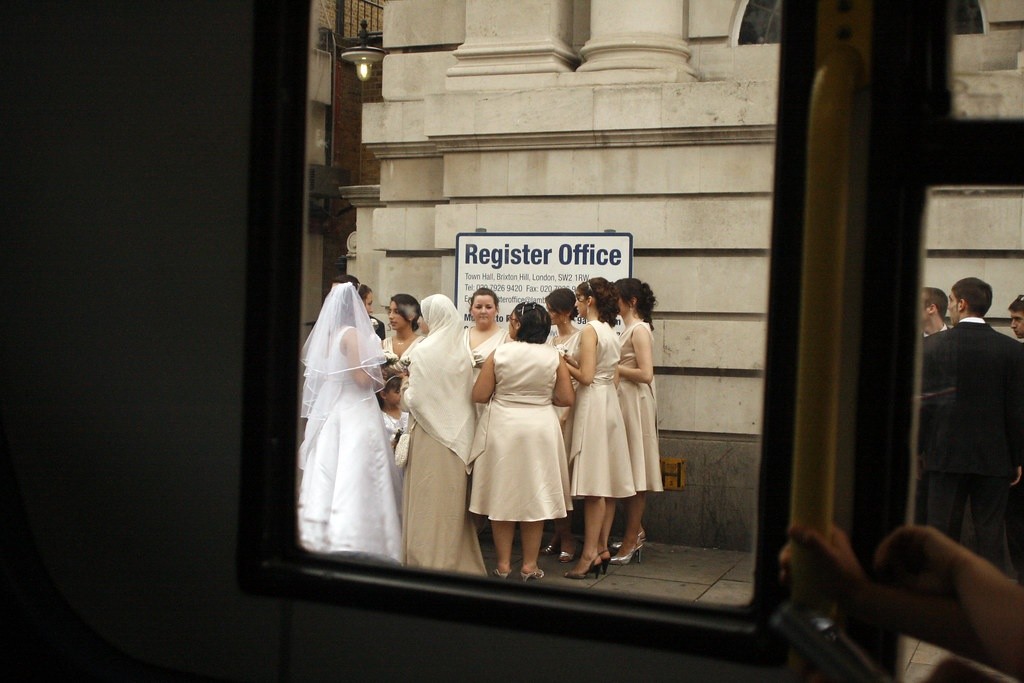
[609,537,643,565]
[493,569,512,579]
[541,543,558,555]
[559,541,578,563]
[610,530,646,559]
[563,554,603,579]
[599,550,611,575]
[520,569,544,583]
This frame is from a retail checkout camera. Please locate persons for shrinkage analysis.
[563,278,636,581]
[921,284,949,336]
[462,288,514,435]
[610,277,663,569]
[916,277,1024,581]
[381,295,426,376]
[378,374,415,469]
[402,294,487,580]
[472,303,572,582]
[329,276,385,347]
[777,521,1024,683]
[298,276,403,564]
[1009,294,1024,339]
[540,287,582,562]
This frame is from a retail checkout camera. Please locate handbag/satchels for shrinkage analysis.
[395,434,410,468]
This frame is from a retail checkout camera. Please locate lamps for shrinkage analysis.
[341,17,386,82]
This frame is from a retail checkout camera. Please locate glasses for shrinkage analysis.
[1017,295,1024,301]
[508,315,516,321]
